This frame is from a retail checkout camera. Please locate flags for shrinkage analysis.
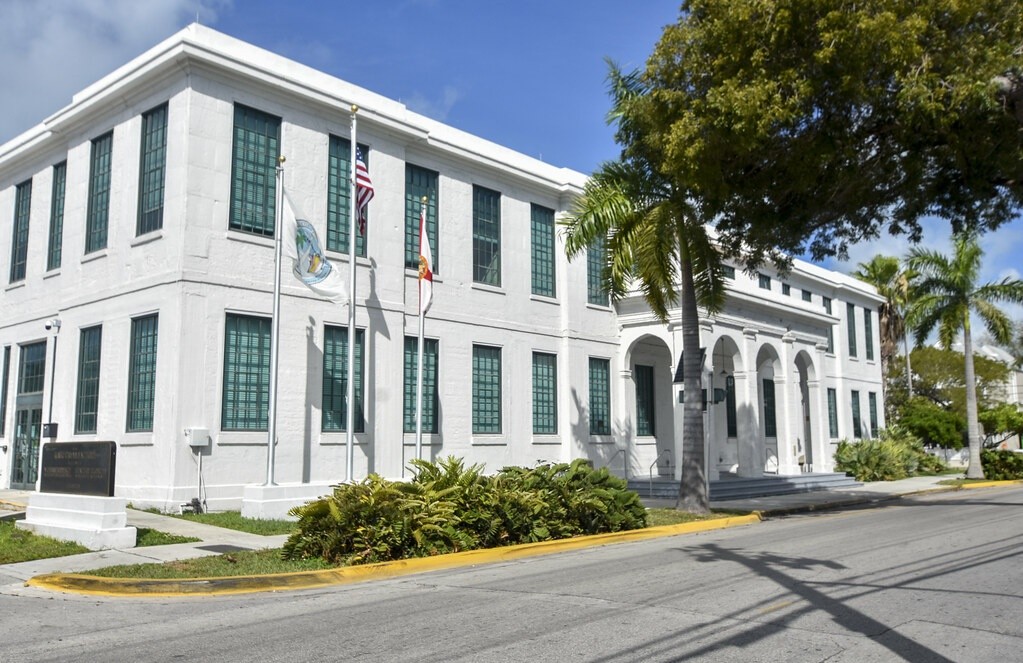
[348,123,374,237]
[418,209,435,332]
[278,175,353,306]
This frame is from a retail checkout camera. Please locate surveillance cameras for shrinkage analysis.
[45,321,52,330]
